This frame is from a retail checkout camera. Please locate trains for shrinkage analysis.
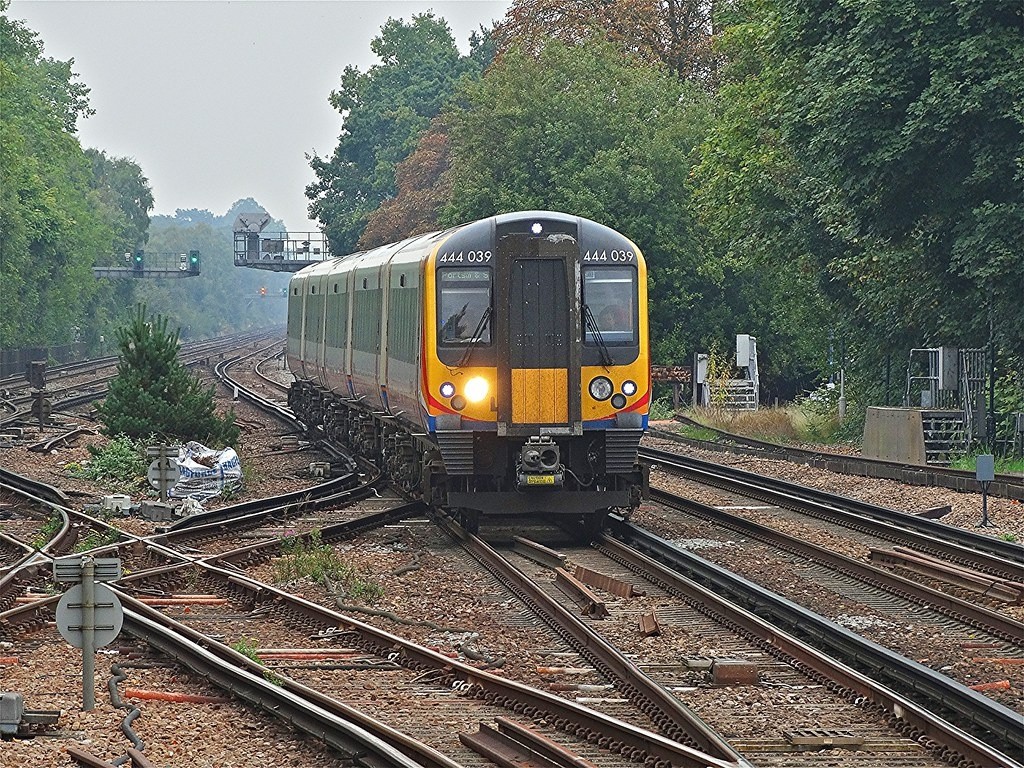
[284,208,653,538]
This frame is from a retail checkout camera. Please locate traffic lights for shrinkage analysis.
[283,288,287,298]
[133,248,144,270]
[190,248,200,272]
[261,287,266,298]
